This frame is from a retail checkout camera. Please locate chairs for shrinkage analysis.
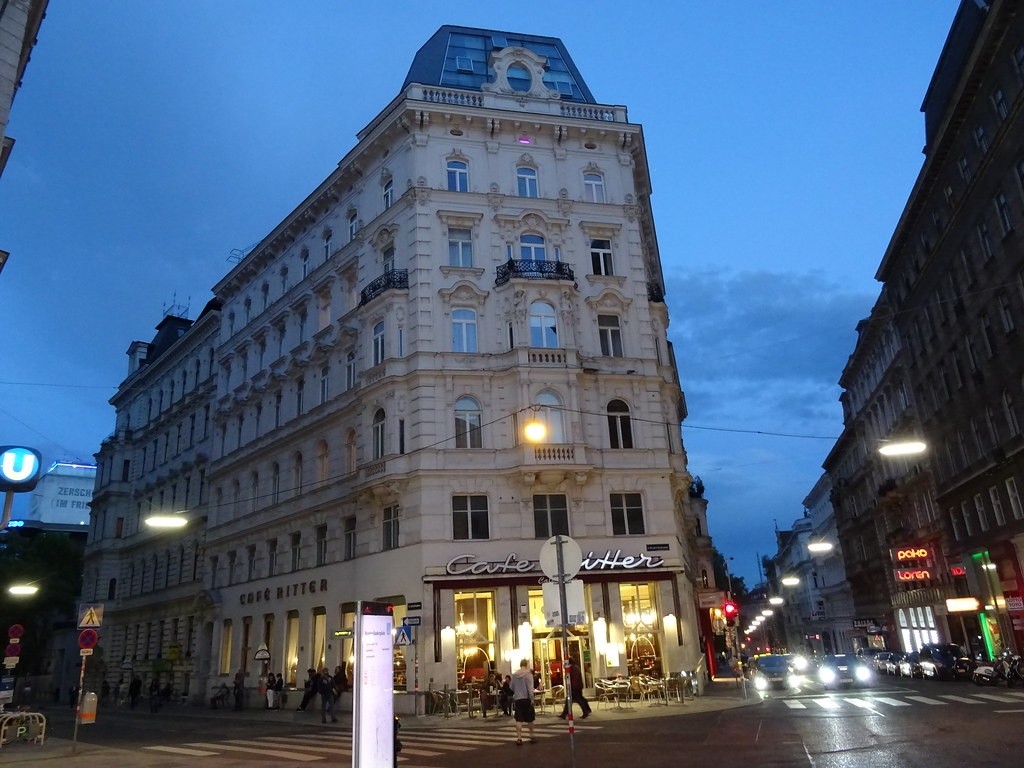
[217,692,230,708]
[429,669,693,718]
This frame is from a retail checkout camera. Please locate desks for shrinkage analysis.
[511,691,546,718]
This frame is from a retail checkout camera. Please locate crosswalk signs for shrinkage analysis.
[76,602,104,630]
[395,626,412,645]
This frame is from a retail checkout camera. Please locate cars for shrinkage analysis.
[745,642,974,690]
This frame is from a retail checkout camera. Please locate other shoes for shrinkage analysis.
[296,706,305,713]
[516,739,523,744]
[278,706,280,711]
[530,737,537,743]
[332,717,337,722]
[273,707,278,711]
[266,707,273,711]
[322,718,326,723]
[557,714,566,720]
[581,710,591,718]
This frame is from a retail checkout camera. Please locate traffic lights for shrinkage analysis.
[724,603,736,626]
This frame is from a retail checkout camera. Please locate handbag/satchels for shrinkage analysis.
[521,703,535,722]
[395,738,402,751]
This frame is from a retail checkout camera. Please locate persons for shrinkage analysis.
[295,666,347,724]
[265,673,283,710]
[70,678,174,714]
[481,660,539,746]
[209,683,229,709]
[740,652,754,680]
[557,657,591,720]
[233,669,244,695]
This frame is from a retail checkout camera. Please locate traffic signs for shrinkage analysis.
[399,616,421,626]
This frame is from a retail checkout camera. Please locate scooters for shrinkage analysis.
[970,647,1024,685]
